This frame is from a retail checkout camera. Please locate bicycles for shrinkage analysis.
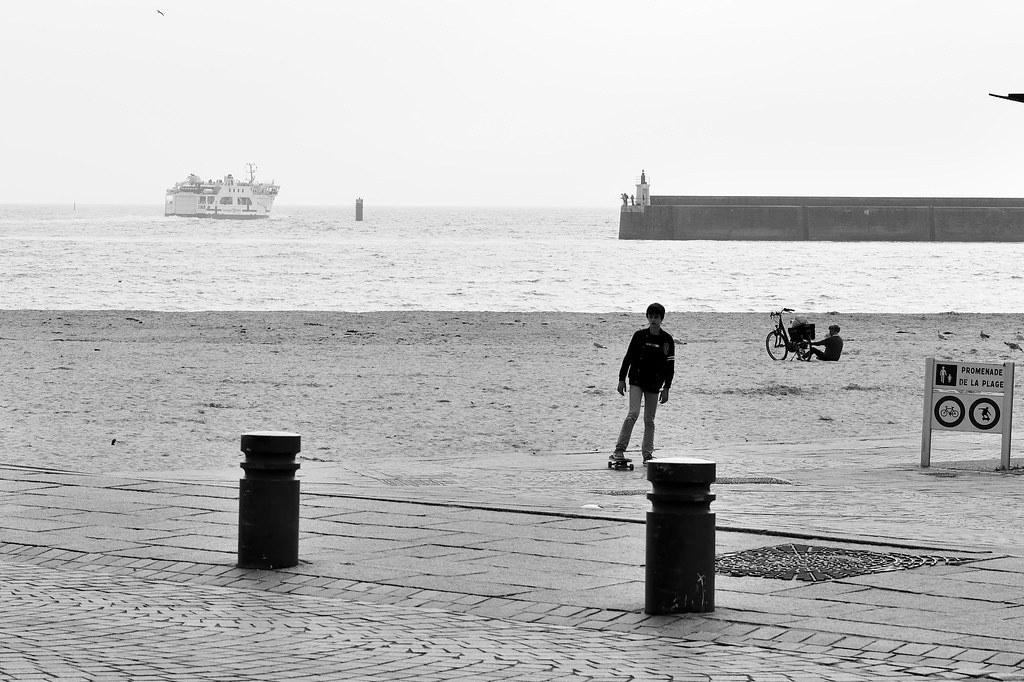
[765,308,813,362]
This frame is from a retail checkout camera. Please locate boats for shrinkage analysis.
[163,162,279,221]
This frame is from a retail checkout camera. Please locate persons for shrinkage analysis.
[795,325,844,362]
[612,302,675,466]
[620,193,635,206]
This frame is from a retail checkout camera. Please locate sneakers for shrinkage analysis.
[642,454,653,466]
[613,449,626,460]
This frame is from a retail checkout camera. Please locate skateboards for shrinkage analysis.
[607,454,634,470]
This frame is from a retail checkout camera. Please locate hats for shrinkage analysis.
[828,324,841,334]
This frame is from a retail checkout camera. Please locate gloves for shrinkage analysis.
[617,381,626,396]
[658,388,669,404]
